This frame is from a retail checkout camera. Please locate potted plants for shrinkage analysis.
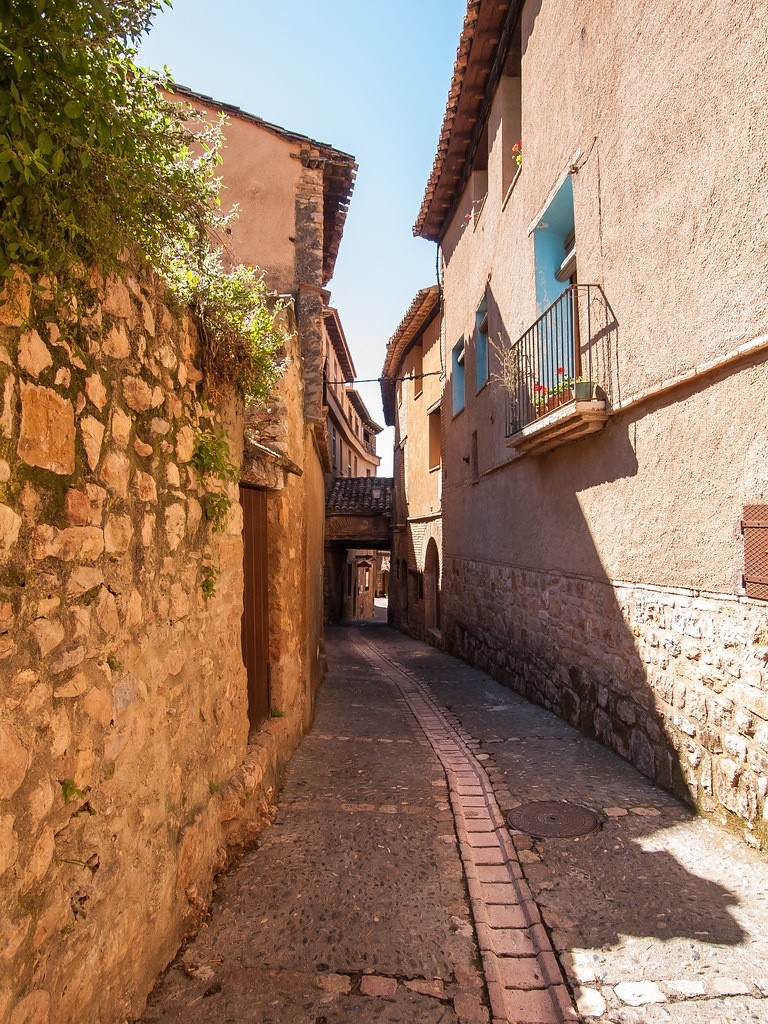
[576,376,596,401]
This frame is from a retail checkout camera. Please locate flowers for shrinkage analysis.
[510,139,523,165]
[532,368,583,405]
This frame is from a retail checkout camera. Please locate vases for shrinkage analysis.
[555,390,574,405]
[536,396,559,417]
